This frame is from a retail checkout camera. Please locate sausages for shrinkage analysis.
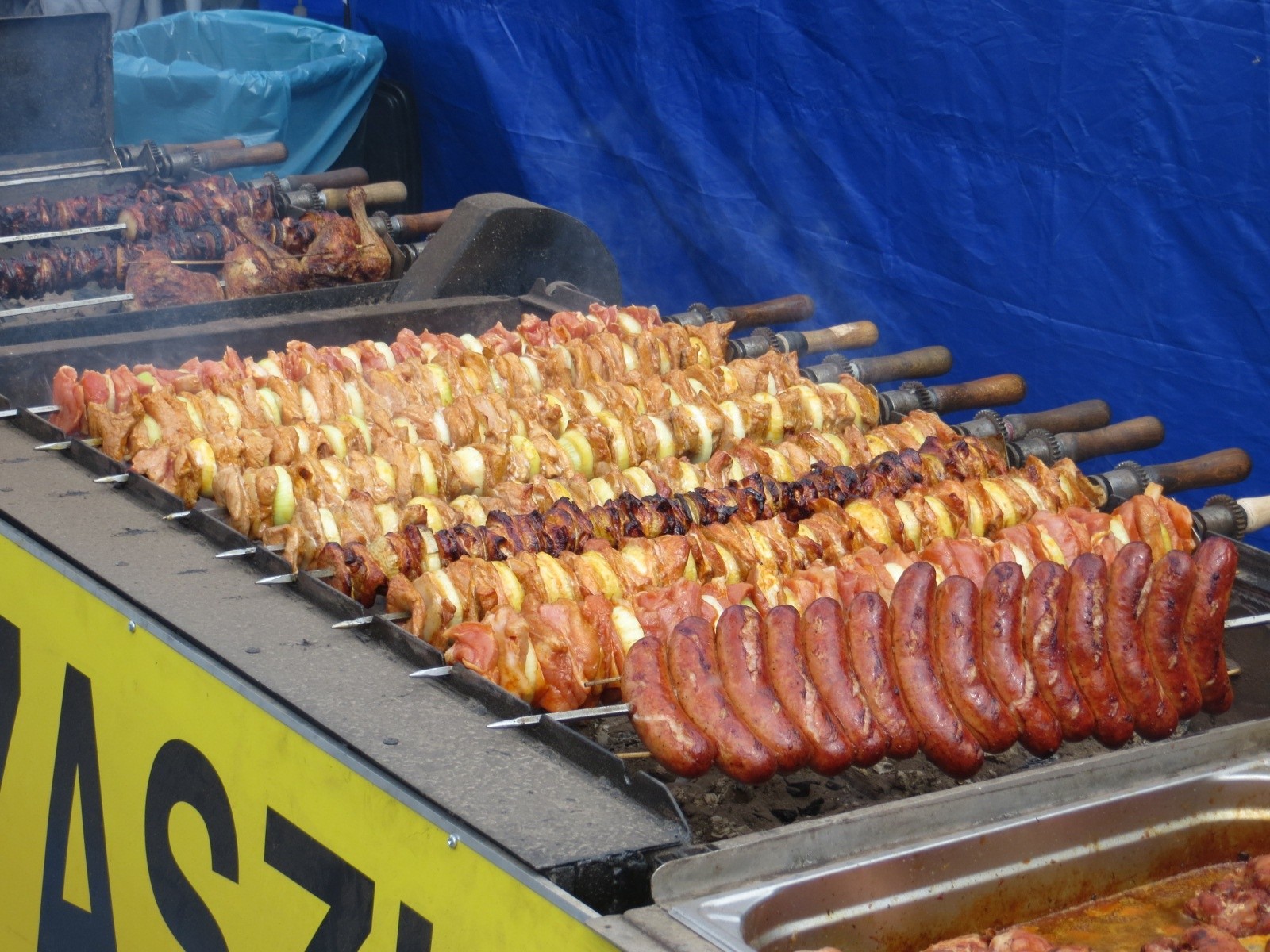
[630,530,1240,778]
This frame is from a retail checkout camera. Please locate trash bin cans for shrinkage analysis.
[109,7,386,179]
